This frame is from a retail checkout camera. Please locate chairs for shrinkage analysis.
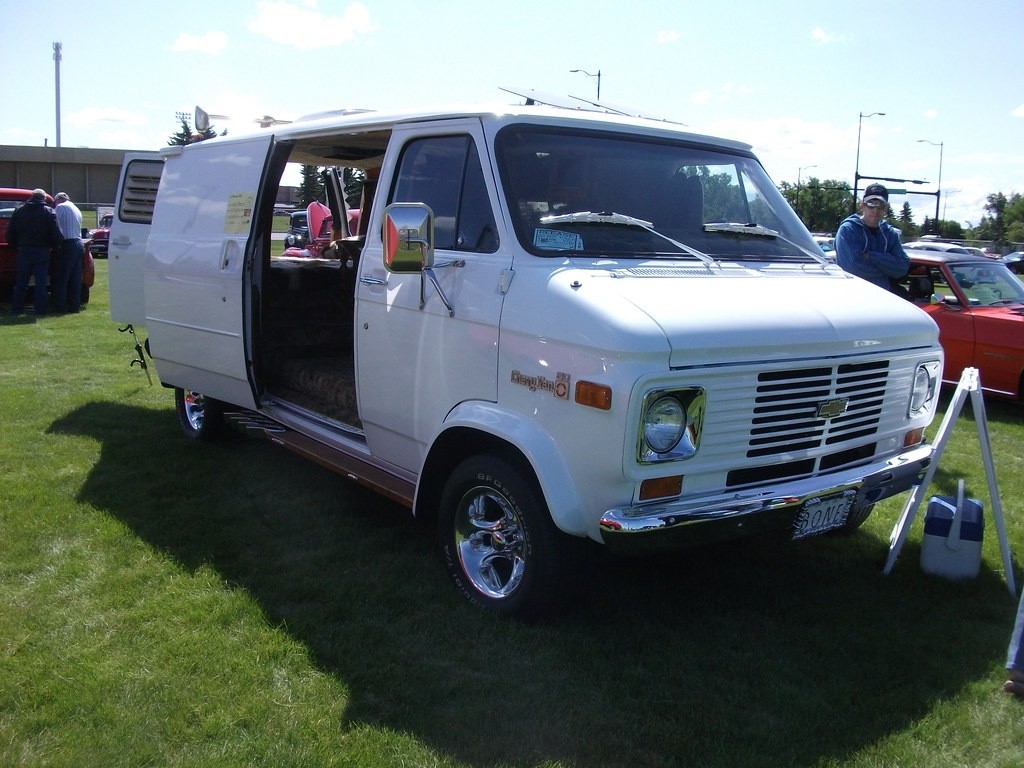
[909,278,931,302]
[459,156,536,246]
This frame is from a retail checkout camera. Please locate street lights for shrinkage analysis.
[853,111,885,214]
[571,70,601,102]
[796,166,818,212]
[943,190,961,231]
[916,139,944,232]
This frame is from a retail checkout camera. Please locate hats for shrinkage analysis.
[52,193,69,209]
[863,182,888,202]
[33,189,46,199]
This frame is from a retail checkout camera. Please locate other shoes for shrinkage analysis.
[5,309,15,313]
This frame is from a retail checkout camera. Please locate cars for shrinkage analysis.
[86,214,113,258]
[285,211,312,251]
[812,231,1024,408]
[282,200,362,258]
[0,187,95,307]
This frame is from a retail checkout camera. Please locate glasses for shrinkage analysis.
[865,201,885,209]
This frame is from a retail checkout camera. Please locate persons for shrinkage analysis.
[4,188,65,316]
[832,183,912,292]
[49,191,85,313]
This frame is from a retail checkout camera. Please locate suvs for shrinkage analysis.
[117,116,943,612]
